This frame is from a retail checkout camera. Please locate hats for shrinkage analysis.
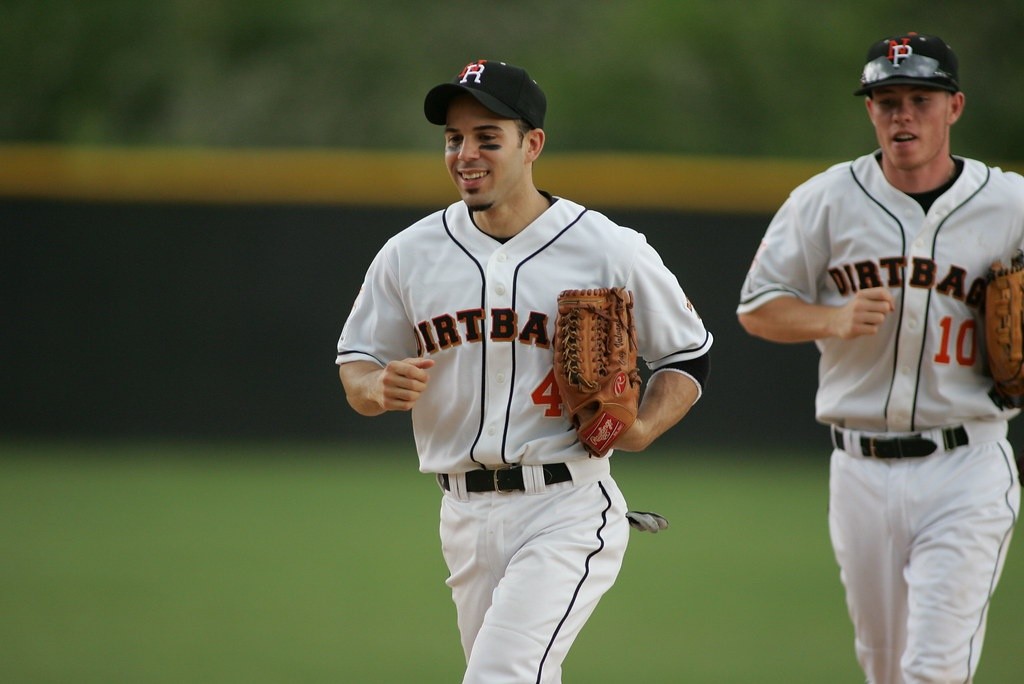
[853,31,960,96]
[423,60,547,129]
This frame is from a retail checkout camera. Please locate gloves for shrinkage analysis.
[626,510,669,533]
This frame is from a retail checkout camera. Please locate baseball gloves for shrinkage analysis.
[969,256,1024,404]
[553,286,640,454]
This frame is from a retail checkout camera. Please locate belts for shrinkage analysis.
[442,462,572,492]
[834,424,970,458]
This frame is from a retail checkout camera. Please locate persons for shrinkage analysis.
[735,27,1023,684]
[335,58,716,684]
